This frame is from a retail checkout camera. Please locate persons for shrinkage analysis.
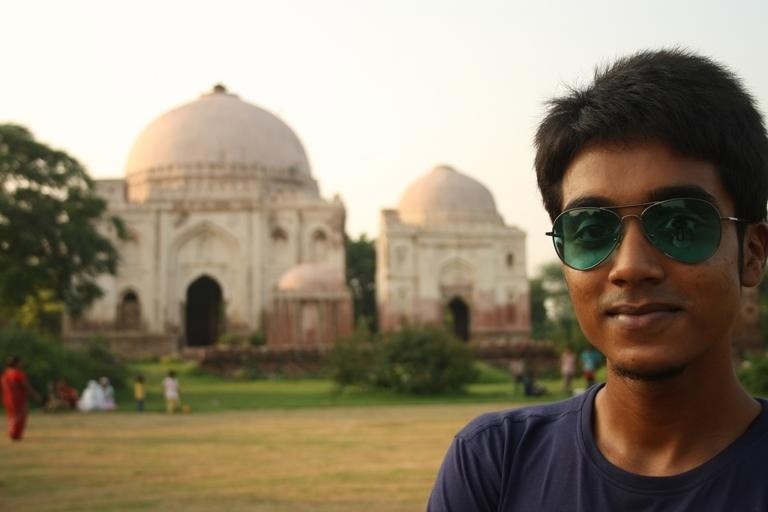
[0,354,43,442]
[425,43,768,512]
[44,373,118,412]
[161,369,181,413]
[510,340,603,396]
[133,374,146,411]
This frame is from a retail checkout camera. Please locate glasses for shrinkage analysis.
[545,198,752,271]
